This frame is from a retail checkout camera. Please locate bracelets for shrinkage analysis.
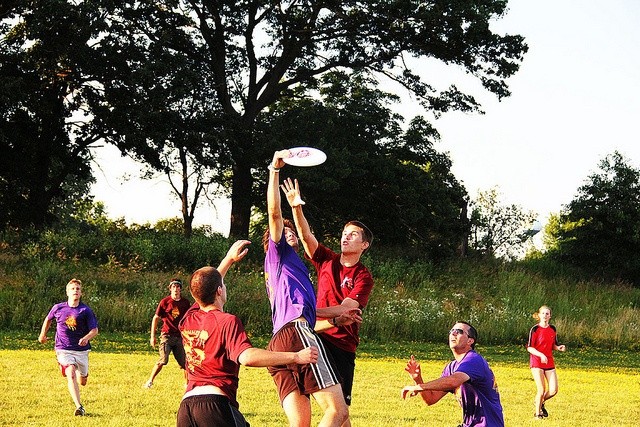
[267,163,280,173]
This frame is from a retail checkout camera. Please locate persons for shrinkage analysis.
[279,175,374,427]
[145,279,191,390]
[527,305,566,419]
[39,278,99,417]
[262,149,349,427]
[175,239,318,427]
[401,320,504,427]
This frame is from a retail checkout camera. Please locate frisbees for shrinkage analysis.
[282,146,328,167]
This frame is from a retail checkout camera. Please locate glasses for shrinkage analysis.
[449,329,470,337]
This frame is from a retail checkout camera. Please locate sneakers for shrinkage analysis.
[540,403,548,417]
[145,382,152,388]
[74,404,84,416]
